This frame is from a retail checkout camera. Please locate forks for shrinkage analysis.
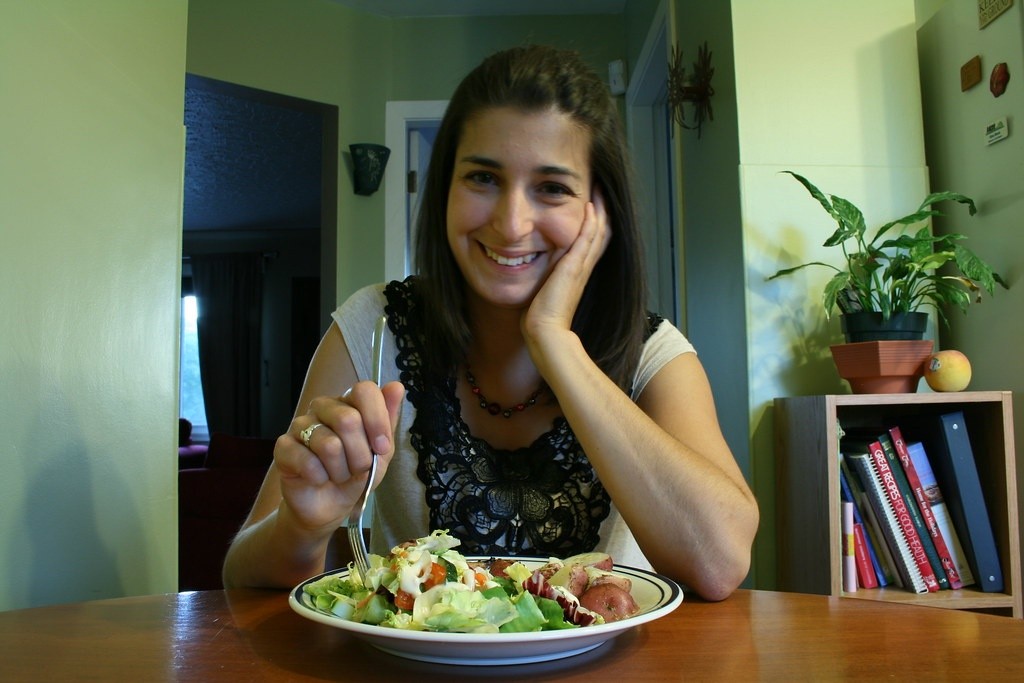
[345,317,389,588]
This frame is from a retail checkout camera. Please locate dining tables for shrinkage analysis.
[0,588,1024,683]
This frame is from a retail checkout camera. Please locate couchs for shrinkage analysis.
[179,467,274,590]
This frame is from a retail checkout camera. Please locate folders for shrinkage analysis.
[883,410,1003,593]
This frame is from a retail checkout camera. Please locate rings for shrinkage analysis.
[298,423,323,447]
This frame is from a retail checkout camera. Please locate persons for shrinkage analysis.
[224,43,764,605]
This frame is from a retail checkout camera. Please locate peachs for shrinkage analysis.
[923,350,972,392]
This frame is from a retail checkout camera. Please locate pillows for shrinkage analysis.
[203,431,274,467]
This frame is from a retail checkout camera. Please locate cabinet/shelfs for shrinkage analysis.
[773,390,1024,618]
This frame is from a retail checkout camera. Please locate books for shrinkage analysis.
[838,424,977,593]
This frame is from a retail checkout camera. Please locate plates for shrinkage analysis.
[287,555,685,667]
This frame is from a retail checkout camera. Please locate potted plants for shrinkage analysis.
[765,170,1010,343]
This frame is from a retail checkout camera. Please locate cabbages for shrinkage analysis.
[300,530,584,634]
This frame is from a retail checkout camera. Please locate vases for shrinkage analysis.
[830,340,934,394]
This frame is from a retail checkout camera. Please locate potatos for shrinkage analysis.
[488,552,640,623]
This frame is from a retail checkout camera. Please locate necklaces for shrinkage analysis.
[457,339,553,420]
